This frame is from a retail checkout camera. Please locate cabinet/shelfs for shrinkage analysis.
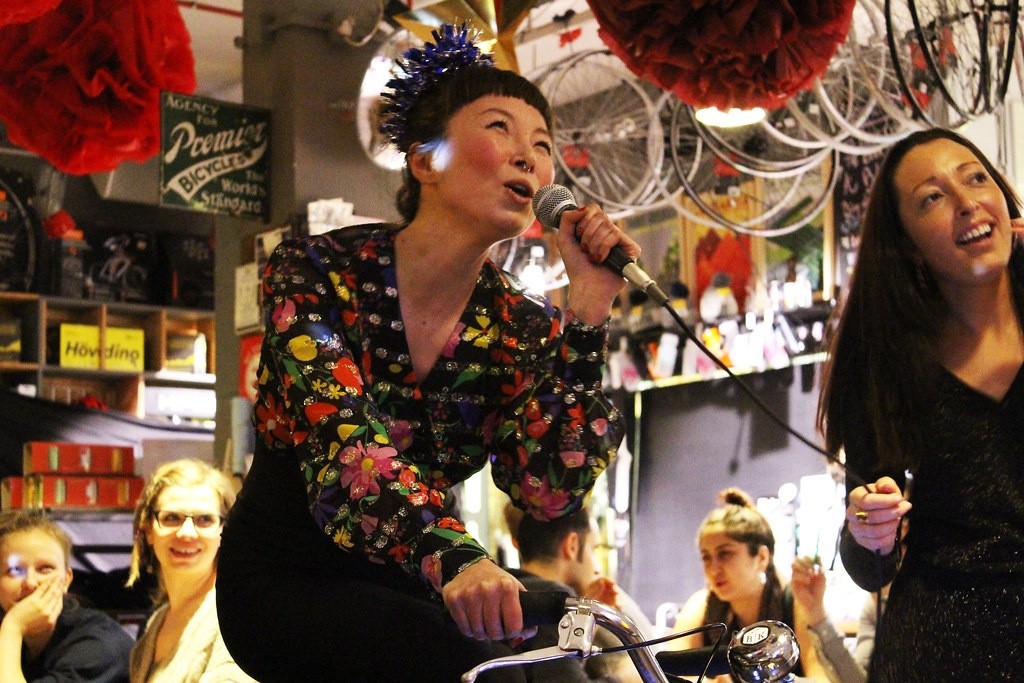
[1,146,241,523]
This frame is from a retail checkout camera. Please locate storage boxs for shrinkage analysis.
[605,148,837,311]
[2,442,143,512]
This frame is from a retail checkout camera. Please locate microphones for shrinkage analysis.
[532,183,669,305]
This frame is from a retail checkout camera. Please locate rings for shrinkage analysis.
[856,511,867,523]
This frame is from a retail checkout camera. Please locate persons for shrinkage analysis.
[128,458,255,683]
[668,488,831,683]
[0,508,135,683]
[506,495,644,683]
[218,66,642,683]
[817,127,1024,683]
[793,555,889,682]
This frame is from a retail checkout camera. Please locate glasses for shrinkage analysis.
[151,509,226,530]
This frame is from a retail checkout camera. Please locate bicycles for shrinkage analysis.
[425,565,805,683]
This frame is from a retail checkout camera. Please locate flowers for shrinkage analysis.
[0,0,198,175]
[588,1,859,108]
